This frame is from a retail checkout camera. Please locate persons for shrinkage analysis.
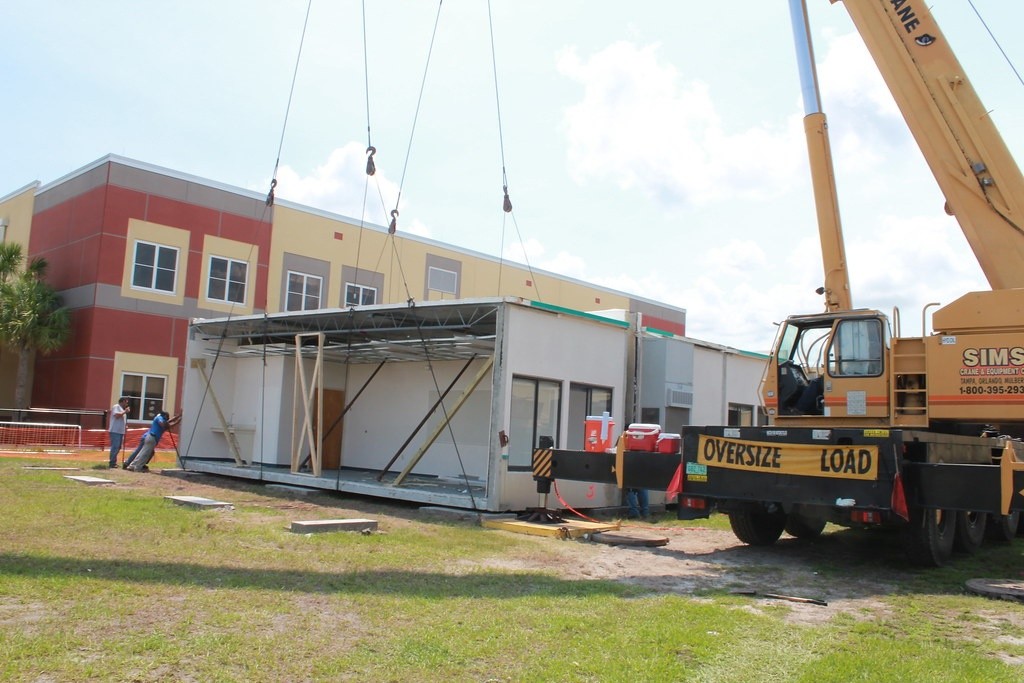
[108,397,130,468]
[123,411,182,472]
[625,487,650,519]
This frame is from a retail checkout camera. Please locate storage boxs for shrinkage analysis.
[626,423,661,452]
[656,433,681,453]
[585,416,616,452]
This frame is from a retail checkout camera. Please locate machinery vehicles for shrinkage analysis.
[181,1,1023,567]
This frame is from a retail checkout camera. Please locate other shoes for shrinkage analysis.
[109,463,118,468]
[123,463,128,468]
[128,468,149,472]
